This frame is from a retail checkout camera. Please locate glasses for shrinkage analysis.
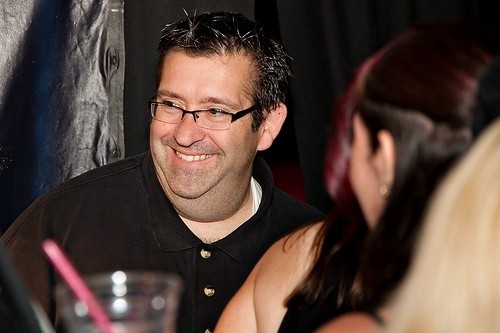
[149,94,262,131]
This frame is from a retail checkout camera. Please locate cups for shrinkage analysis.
[56,271,182,333]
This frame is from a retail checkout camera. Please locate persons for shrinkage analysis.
[214,37,500,333]
[0,7,322,333]
